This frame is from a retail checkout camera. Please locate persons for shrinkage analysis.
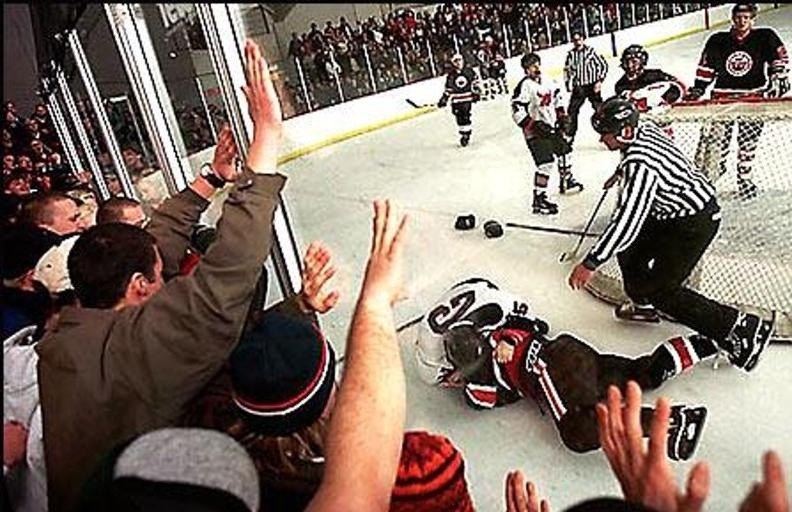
[563,96,763,371]
[614,43,689,148]
[413,275,537,390]
[309,4,722,111]
[594,379,792,511]
[680,2,792,199]
[504,470,660,512]
[1,34,417,512]
[510,52,585,211]
[437,53,483,150]
[448,313,734,463]
[560,29,623,151]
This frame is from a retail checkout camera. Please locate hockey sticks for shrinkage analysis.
[560,173,618,263]
[405,98,438,110]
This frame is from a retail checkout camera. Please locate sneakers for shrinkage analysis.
[533,190,557,209]
[616,300,657,319]
[728,312,761,366]
[665,405,687,460]
[560,173,581,192]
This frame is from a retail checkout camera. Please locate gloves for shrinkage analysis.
[683,86,703,101]
[484,221,503,238]
[533,121,551,138]
[762,73,790,99]
[456,215,476,229]
[559,116,572,133]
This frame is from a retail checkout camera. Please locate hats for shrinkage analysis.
[227,314,334,438]
[2,219,59,281]
[92,427,261,512]
[32,234,78,301]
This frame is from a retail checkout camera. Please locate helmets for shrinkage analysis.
[732,1,758,18]
[590,95,640,143]
[620,44,648,74]
[446,326,482,366]
